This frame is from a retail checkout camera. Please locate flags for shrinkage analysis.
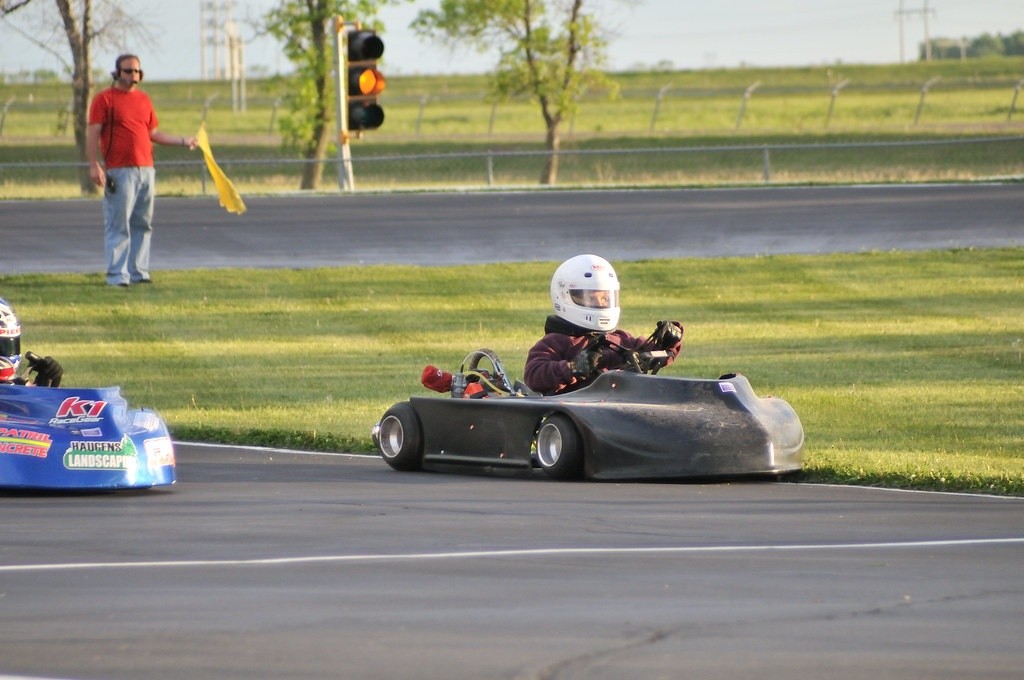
[195,128,247,215]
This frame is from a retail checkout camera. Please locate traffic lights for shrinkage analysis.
[346,27,386,132]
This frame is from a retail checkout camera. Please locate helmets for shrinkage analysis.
[0,298,28,383]
[551,254,621,334]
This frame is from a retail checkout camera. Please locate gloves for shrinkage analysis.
[31,355,63,387]
[655,320,681,351]
[570,348,602,378]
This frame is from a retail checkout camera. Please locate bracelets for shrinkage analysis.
[182,138,185,146]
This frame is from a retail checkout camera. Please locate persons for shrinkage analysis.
[86,54,198,286]
[524,253,683,396]
[0,297,63,388]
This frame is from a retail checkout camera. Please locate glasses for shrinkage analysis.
[120,68,141,75]
